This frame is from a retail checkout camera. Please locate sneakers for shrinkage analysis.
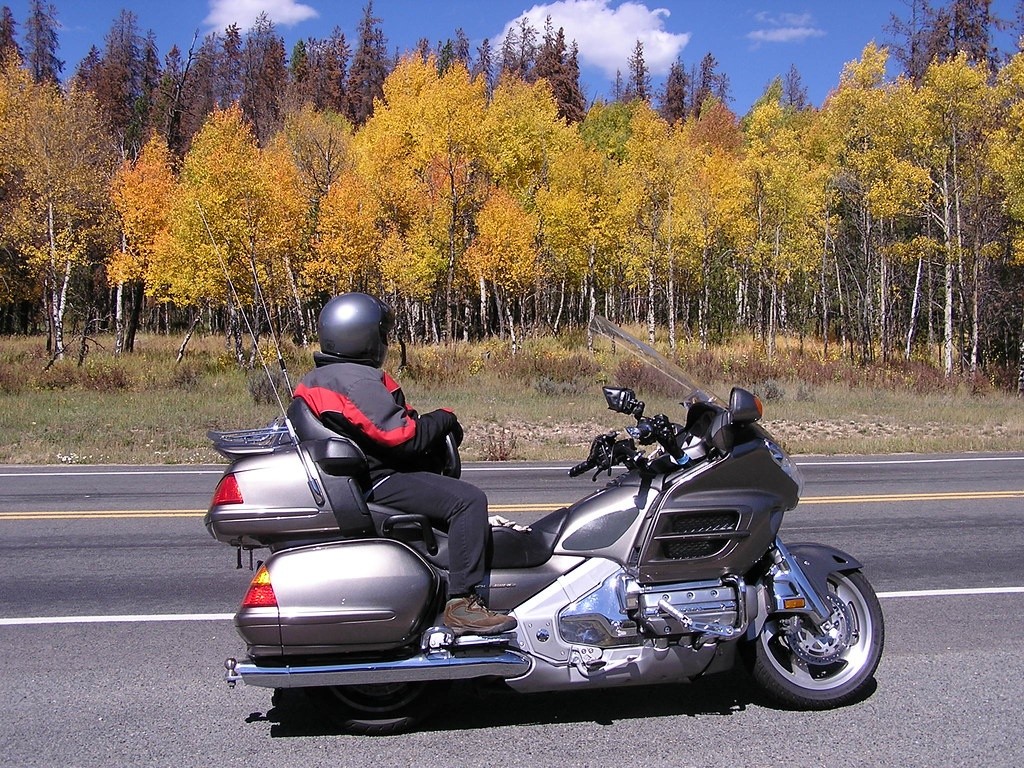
[445,596,518,640]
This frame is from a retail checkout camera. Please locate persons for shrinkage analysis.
[291,292,516,636]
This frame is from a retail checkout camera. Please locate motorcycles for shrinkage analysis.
[199,313,885,738]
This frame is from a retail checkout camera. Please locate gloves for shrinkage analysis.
[449,418,462,448]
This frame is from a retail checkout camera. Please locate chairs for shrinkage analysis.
[287,399,461,571]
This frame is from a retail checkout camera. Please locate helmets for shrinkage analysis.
[317,291,399,365]
